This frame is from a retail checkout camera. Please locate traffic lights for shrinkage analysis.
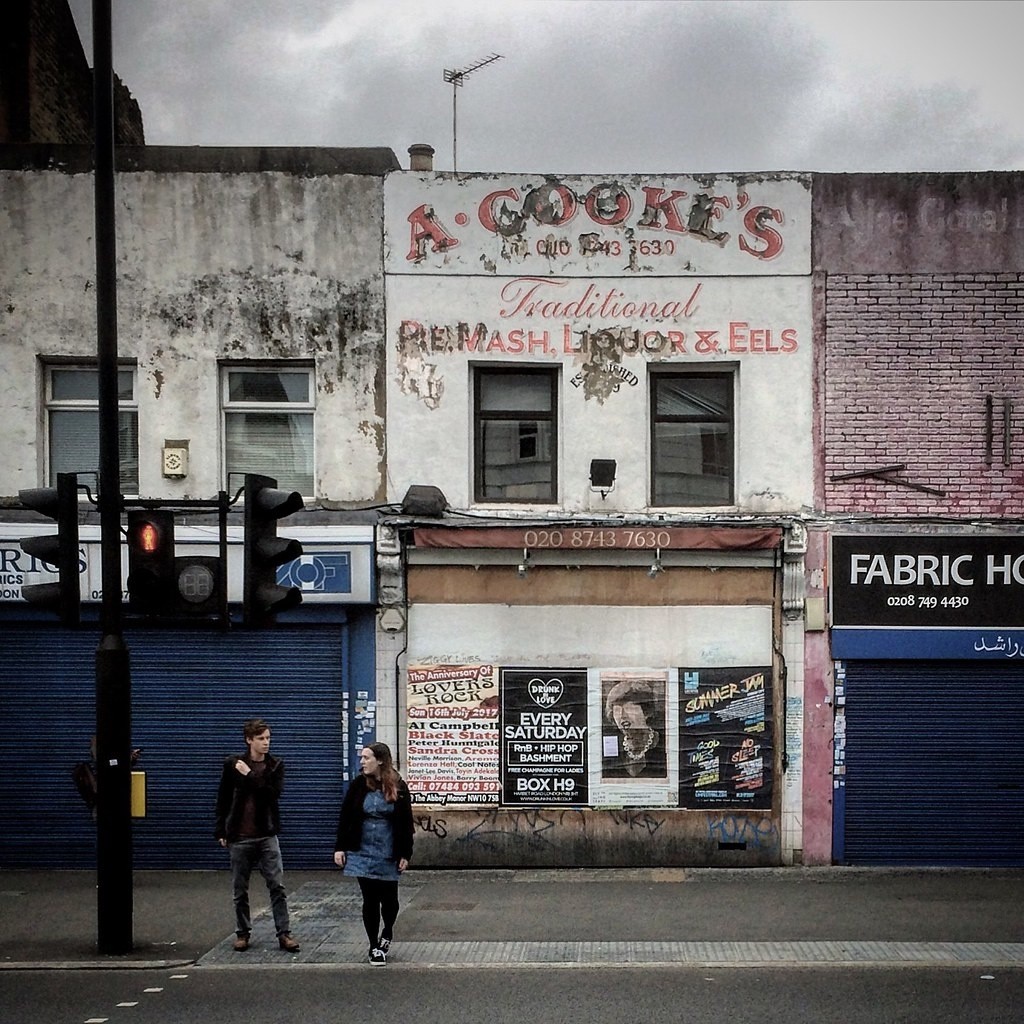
[15,471,80,630]
[126,509,172,616]
[243,472,303,621]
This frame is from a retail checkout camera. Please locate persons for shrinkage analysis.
[215,720,300,951]
[603,681,665,777]
[89,736,140,822]
[333,743,415,967]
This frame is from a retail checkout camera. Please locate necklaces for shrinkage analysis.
[623,726,656,760]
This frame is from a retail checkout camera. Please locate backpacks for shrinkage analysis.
[73,761,99,807]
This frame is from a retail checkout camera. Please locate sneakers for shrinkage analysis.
[278,934,300,950]
[379,928,393,955]
[234,937,249,951]
[367,946,387,966]
